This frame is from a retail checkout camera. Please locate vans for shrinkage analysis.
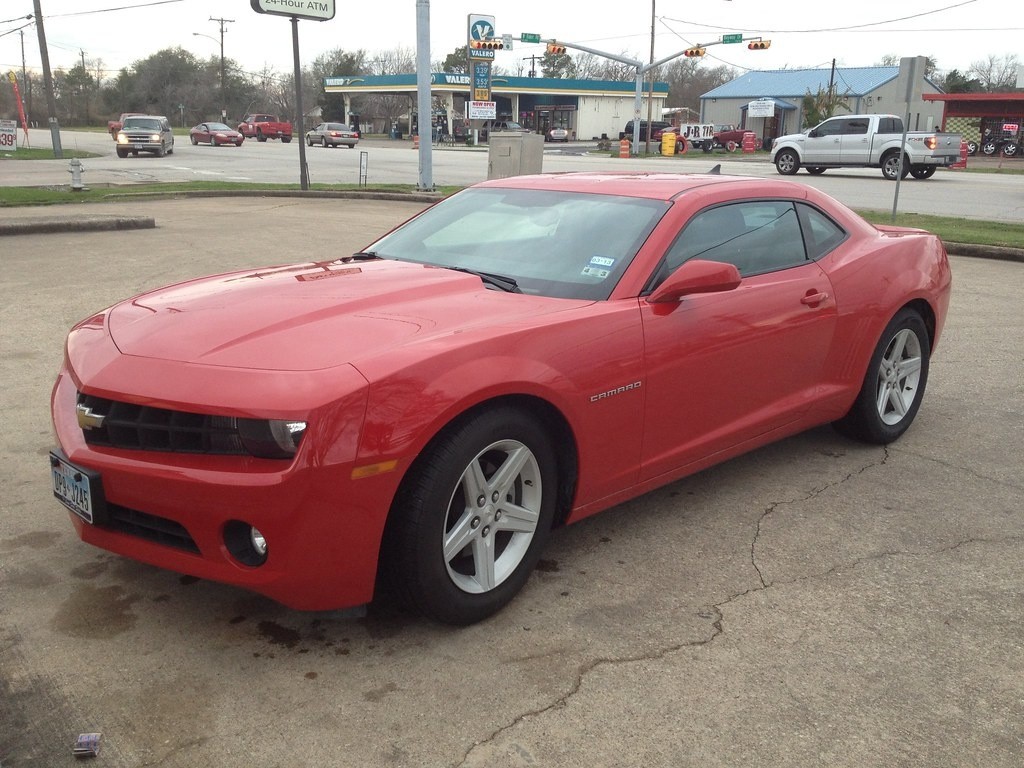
[624,120,674,141]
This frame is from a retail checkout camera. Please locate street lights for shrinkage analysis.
[192,32,229,126]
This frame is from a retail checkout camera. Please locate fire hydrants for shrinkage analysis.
[66,157,101,198]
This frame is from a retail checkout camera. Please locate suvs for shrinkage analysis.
[115,115,174,157]
[481,119,532,137]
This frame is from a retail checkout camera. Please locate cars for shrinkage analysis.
[653,127,682,142]
[545,125,568,143]
[189,122,244,148]
[305,122,360,148]
[441,118,472,138]
[983,134,1024,156]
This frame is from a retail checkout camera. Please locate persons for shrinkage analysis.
[935,126,941,132]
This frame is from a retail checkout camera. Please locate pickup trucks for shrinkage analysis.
[108,113,148,141]
[712,124,753,147]
[769,114,961,180]
[237,114,293,144]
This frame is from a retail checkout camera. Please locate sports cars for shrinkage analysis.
[47,162,954,622]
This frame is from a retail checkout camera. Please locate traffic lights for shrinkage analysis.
[470,39,504,50]
[548,44,566,54]
[684,49,705,56]
[747,42,769,50]
